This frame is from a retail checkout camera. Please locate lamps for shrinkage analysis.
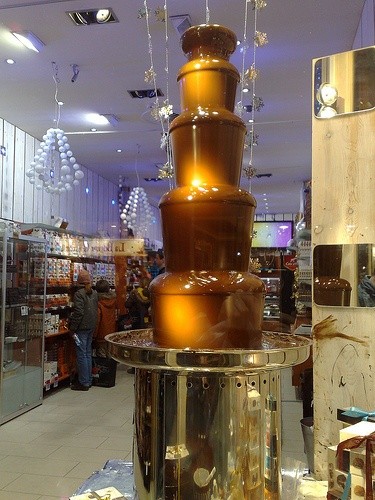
[14,32,46,53]
[119,144,156,235]
[25,62,84,195]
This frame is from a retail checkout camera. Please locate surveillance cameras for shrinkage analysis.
[68,65,81,82]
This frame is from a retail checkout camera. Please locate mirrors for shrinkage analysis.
[313,47,375,120]
[312,243,375,309]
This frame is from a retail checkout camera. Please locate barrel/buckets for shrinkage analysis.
[299,417,315,474]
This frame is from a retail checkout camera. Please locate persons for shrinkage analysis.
[290,306,314,419]
[278,267,298,335]
[92,278,118,388]
[122,276,152,329]
[67,269,100,391]
[146,252,158,279]
[155,248,167,276]
[357,271,375,308]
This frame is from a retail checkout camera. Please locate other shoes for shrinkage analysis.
[127,369,135,374]
[71,385,89,391]
[74,381,92,387]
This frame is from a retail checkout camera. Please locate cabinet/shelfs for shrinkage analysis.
[0,233,48,425]
[15,228,150,389]
[248,179,313,401]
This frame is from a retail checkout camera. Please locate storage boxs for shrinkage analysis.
[325,406,375,500]
[69,486,129,500]
